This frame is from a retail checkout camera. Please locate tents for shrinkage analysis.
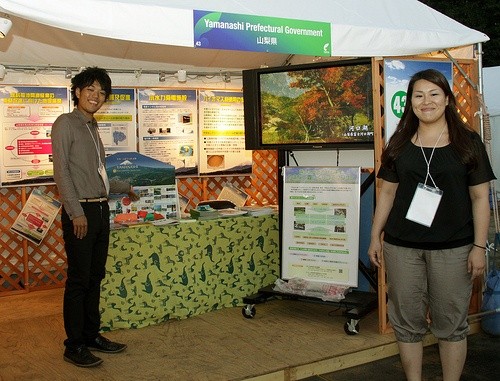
[0,0,490,142]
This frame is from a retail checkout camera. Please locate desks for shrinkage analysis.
[99,207,280,333]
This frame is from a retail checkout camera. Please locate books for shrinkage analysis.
[190,208,218,220]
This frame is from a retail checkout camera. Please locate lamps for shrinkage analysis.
[65,69,71,79]
[0,64,7,79]
[159,72,166,81]
[225,73,231,81]
[178,70,186,81]
[0,12,12,38]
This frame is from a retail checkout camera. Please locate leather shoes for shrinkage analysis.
[87,334,127,353]
[63,347,103,368]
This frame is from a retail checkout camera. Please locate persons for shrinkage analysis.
[368,70,497,381]
[51,66,128,367]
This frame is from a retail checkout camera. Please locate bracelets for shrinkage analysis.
[473,243,486,249]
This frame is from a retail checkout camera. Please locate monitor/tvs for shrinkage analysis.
[242,57,375,150]
